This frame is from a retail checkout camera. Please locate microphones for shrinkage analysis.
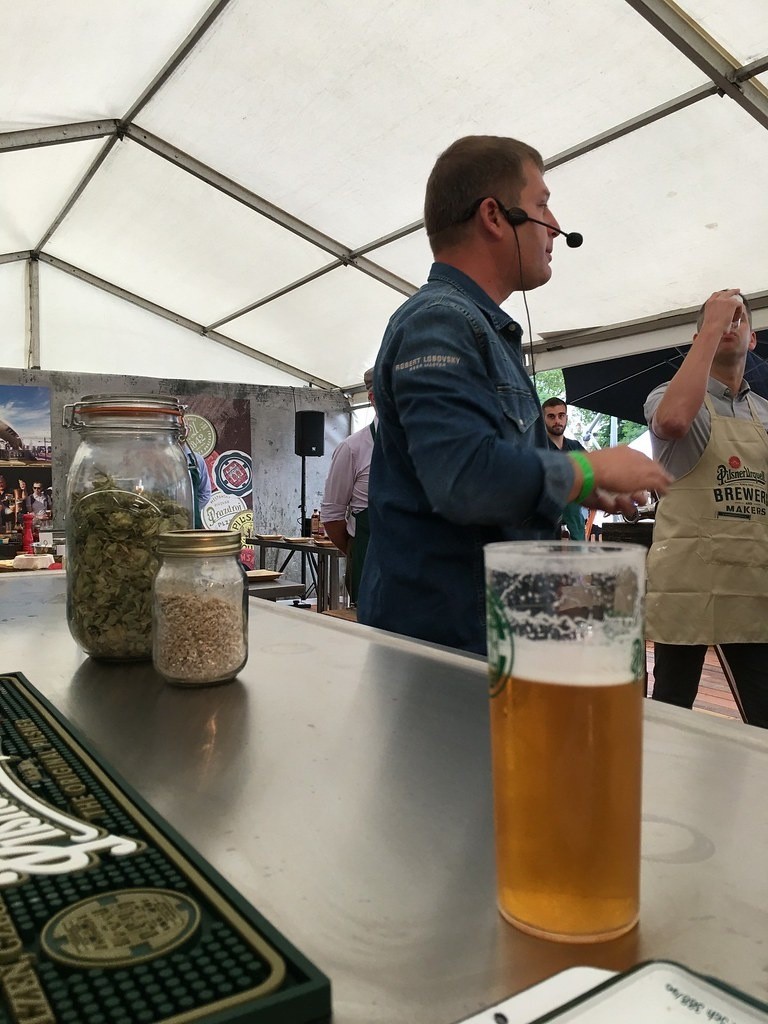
[508,207,584,248]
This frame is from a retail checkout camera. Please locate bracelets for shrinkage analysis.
[567,449,596,504]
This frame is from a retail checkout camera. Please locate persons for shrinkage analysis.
[175,415,212,532]
[0,475,28,528]
[641,287,768,734]
[360,135,675,660]
[543,397,587,541]
[26,480,53,519]
[320,365,381,612]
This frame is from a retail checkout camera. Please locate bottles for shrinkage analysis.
[151,529,251,687]
[63,393,196,658]
[318,511,325,536]
[311,509,319,536]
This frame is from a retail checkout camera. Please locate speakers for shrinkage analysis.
[295,410,324,457]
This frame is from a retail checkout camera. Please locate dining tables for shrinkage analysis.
[3,569,768,1024]
[245,534,354,613]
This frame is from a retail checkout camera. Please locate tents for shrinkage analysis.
[2,0,768,380]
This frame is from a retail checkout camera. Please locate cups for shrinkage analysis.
[482,538,648,945]
